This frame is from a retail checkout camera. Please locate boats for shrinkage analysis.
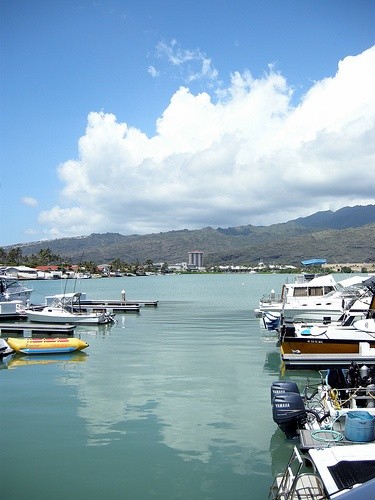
[4,337,89,354]
[0,277,34,314]
[23,298,118,327]
[259,276,375,331]
[16,271,91,279]
[276,319,375,370]
[254,273,341,318]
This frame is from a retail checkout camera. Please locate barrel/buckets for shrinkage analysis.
[344,411,375,442]
